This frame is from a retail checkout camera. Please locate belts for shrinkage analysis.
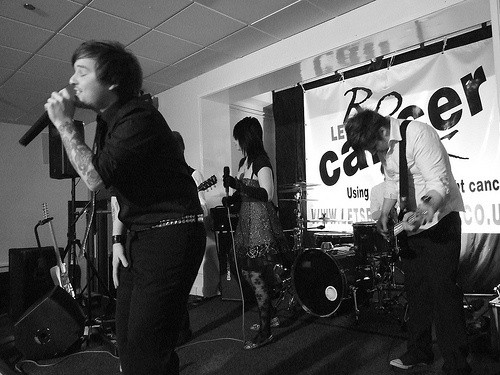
[135,214,203,231]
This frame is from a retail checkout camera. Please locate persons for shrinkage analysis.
[345,110,472,375]
[221,116,281,349]
[43,40,206,375]
[368,162,398,221]
[171,129,209,347]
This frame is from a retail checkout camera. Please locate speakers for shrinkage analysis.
[193,223,247,300]
[7,245,66,307]
[15,285,86,363]
[48,117,86,181]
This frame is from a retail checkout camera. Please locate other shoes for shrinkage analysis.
[244,334,273,350]
[389,351,429,369]
[250,317,280,330]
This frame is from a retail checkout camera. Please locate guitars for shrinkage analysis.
[197,174,219,193]
[41,201,76,299]
[380,205,430,266]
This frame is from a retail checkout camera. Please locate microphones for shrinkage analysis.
[224,166,231,193]
[19,108,53,147]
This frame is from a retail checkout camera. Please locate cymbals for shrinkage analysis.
[279,198,319,202]
[302,217,352,223]
[275,183,321,188]
[278,188,314,193]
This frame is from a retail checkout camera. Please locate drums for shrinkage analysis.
[352,220,380,258]
[291,243,375,318]
[313,231,354,248]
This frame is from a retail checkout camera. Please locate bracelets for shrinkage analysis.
[112,235,123,245]
